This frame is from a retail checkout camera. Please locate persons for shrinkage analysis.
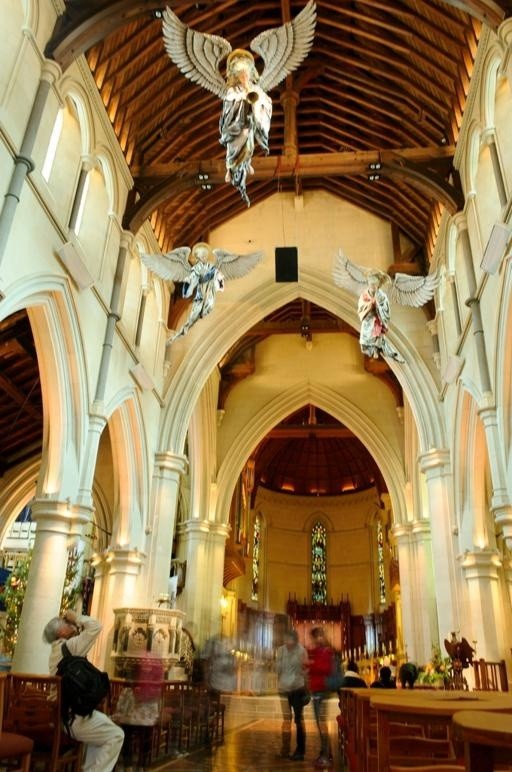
[330,245,441,366]
[301,625,341,768]
[338,660,419,710]
[138,247,262,347]
[269,630,309,762]
[161,0,317,209]
[42,611,125,772]
[113,629,159,772]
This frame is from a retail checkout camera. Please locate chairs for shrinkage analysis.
[1,666,231,771]
[331,677,511,771]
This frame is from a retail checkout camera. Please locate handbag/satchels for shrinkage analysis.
[288,687,310,706]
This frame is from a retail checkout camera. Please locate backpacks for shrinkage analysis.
[55,655,110,716]
